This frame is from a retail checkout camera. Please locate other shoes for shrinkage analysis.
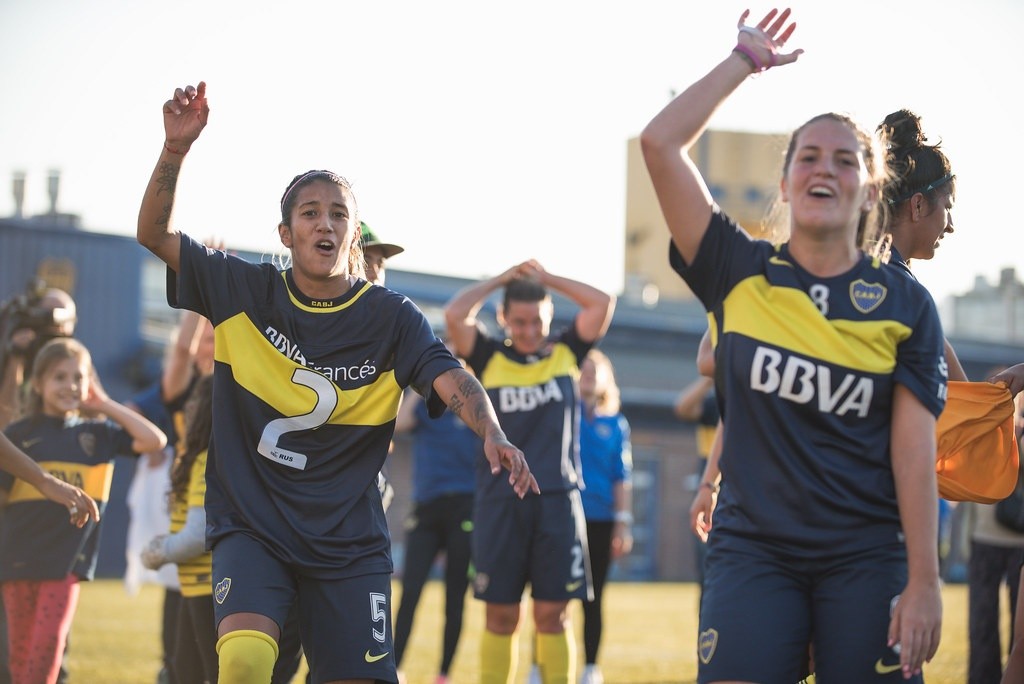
[581,663,603,684]
[529,665,541,684]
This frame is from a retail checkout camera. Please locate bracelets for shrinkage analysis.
[698,482,720,495]
[733,45,762,74]
[613,512,634,526]
[738,27,775,71]
[164,144,186,155]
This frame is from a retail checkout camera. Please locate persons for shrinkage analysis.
[967,363,1024,684]
[0,222,633,684]
[138,82,540,684]
[641,7,969,684]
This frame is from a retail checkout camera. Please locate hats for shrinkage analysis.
[356,222,404,259]
